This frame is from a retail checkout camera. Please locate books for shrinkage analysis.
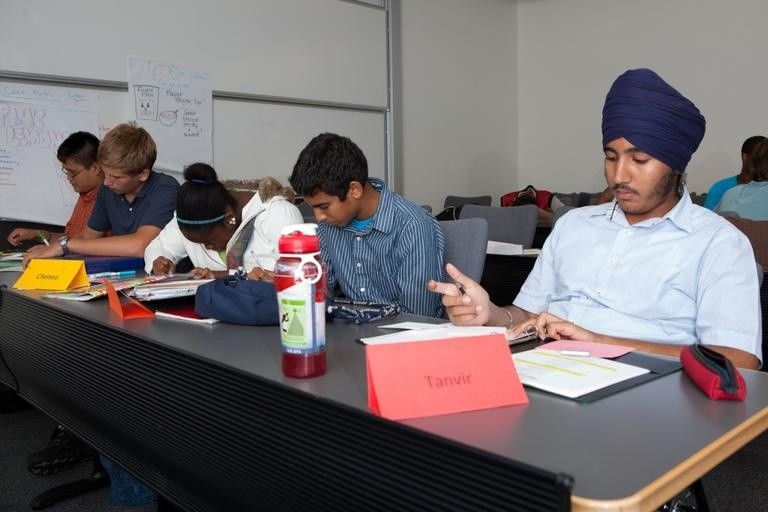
[154,297,222,325]
[486,239,543,256]
[124,268,216,301]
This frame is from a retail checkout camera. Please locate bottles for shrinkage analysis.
[271,223,329,380]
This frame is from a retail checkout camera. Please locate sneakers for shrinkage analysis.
[29,424,94,476]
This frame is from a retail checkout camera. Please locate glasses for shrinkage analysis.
[61,164,92,178]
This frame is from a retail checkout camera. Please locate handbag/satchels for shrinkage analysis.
[194,272,337,328]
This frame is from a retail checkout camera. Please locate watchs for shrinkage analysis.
[59,234,70,257]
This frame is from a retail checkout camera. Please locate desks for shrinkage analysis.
[0,256,768,510]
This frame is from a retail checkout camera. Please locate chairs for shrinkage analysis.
[296,178,709,326]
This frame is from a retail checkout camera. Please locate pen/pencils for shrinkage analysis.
[251,251,265,272]
[87,270,136,277]
[158,237,172,277]
[454,282,465,295]
[37,230,50,246]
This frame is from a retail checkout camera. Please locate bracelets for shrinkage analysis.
[500,307,516,332]
[36,231,51,244]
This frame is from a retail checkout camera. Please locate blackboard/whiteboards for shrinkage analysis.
[0,0,390,112]
[0,75,388,228]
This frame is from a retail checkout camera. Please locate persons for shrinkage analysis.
[7,129,105,246]
[426,69,768,511]
[500,184,565,223]
[702,136,768,212]
[243,131,446,321]
[709,139,768,223]
[141,162,306,286]
[19,120,178,479]
